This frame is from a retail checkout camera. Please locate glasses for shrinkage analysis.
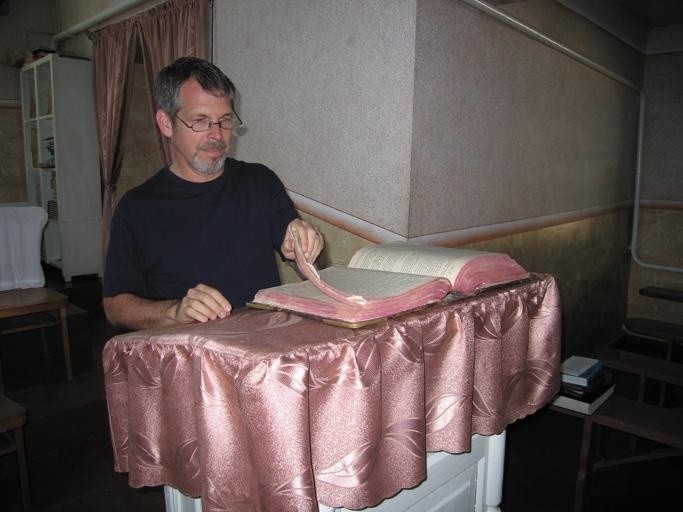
[175,108,242,132]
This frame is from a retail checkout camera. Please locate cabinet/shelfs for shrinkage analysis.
[20,53,102,280]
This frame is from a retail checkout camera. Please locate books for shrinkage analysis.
[560,355,599,376]
[245,224,532,329]
[561,359,603,387]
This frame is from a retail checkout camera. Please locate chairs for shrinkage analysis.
[0,203,73,381]
[0,394,31,512]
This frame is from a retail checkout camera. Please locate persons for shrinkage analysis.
[102,56,326,331]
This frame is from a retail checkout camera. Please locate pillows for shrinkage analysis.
[0,207,48,292]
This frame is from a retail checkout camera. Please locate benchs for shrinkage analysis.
[548,285,683,512]
